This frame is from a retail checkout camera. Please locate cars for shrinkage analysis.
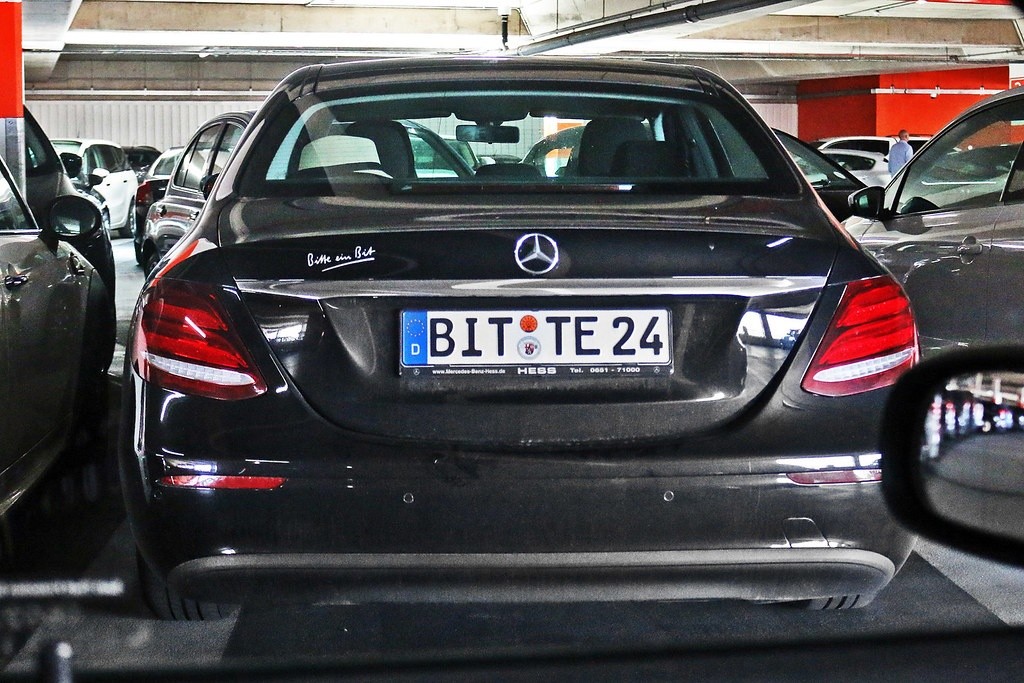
[841,81,1024,355]
[112,51,927,624]
[27,137,138,239]
[122,110,1024,329]
[918,368,1024,474]
[0,96,120,530]
[737,113,1022,240]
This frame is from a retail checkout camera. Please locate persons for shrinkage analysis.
[888,130,913,178]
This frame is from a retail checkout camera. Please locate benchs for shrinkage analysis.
[299,135,685,180]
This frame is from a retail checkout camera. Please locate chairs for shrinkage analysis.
[339,120,416,178]
[577,118,649,173]
